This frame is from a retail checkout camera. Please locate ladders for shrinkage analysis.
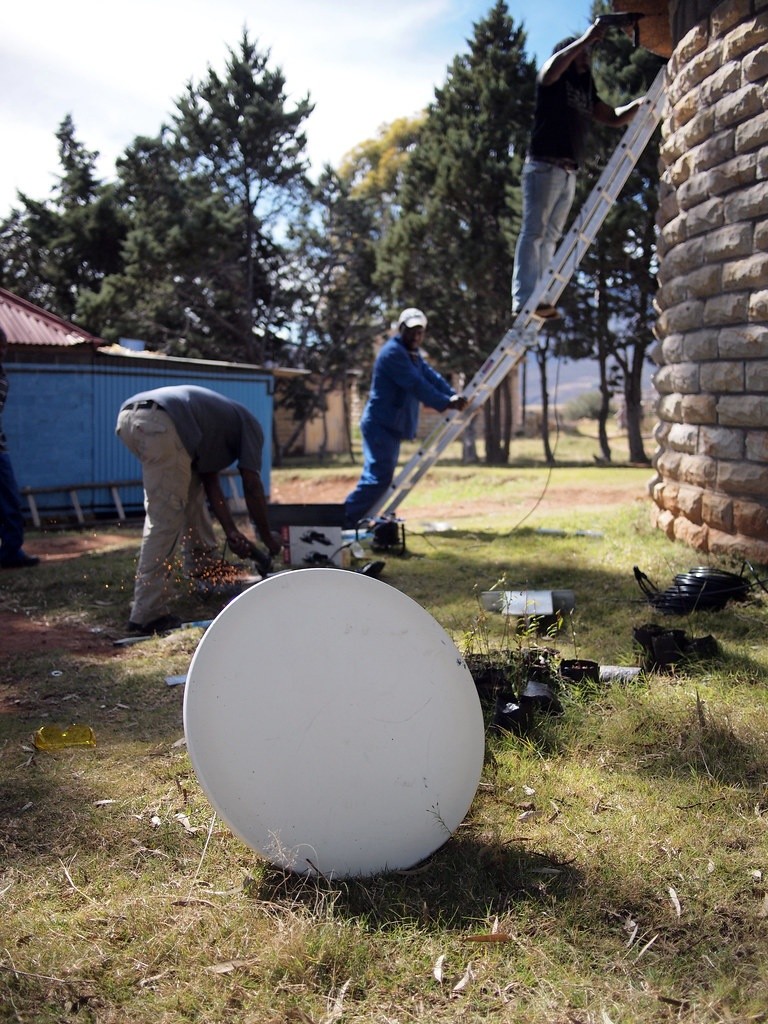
[20,465,272,531]
[358,64,670,526]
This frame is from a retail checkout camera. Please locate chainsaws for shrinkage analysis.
[233,531,285,580]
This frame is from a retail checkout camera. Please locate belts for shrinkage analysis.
[125,400,164,410]
[533,156,578,172]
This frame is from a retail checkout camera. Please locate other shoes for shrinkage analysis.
[341,519,399,535]
[196,563,244,579]
[0,554,39,567]
[511,304,562,321]
[129,615,181,634]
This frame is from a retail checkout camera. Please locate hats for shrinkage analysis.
[398,308,427,331]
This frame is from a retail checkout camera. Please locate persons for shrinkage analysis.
[0,324,44,570]
[510,16,651,317]
[116,385,283,632]
[343,308,468,526]
